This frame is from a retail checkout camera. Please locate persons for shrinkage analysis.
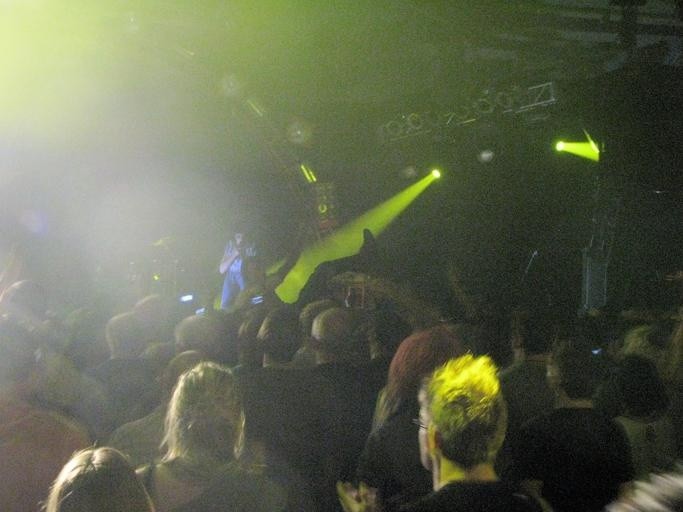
[217,229,259,312]
[0,273,682,512]
